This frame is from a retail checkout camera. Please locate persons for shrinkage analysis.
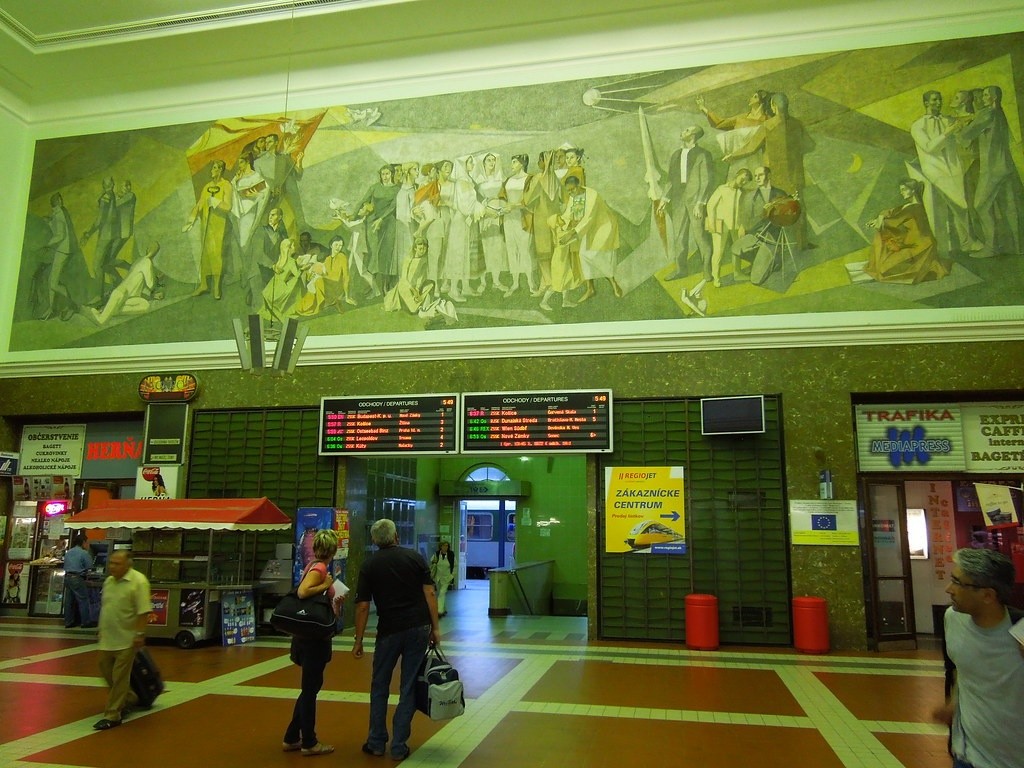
[428,540,457,618]
[928,545,1023,768]
[3,570,22,603]
[280,528,346,757]
[352,517,442,762]
[89,547,156,730]
[150,473,167,499]
[60,536,95,628]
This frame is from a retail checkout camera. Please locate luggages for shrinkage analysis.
[126,647,163,710]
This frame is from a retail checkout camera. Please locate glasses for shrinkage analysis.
[950,578,983,592]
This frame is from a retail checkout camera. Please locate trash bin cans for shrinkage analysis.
[792,596,830,655]
[685,594,719,650]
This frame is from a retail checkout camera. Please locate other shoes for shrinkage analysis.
[435,609,446,620]
[65,620,95,628]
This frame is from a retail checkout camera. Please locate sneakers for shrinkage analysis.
[393,745,412,762]
[362,741,387,758]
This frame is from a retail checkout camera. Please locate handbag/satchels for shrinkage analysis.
[270,593,341,639]
[422,644,464,720]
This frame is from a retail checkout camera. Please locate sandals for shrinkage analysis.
[92,718,121,730]
[280,741,304,751]
[302,742,335,756]
